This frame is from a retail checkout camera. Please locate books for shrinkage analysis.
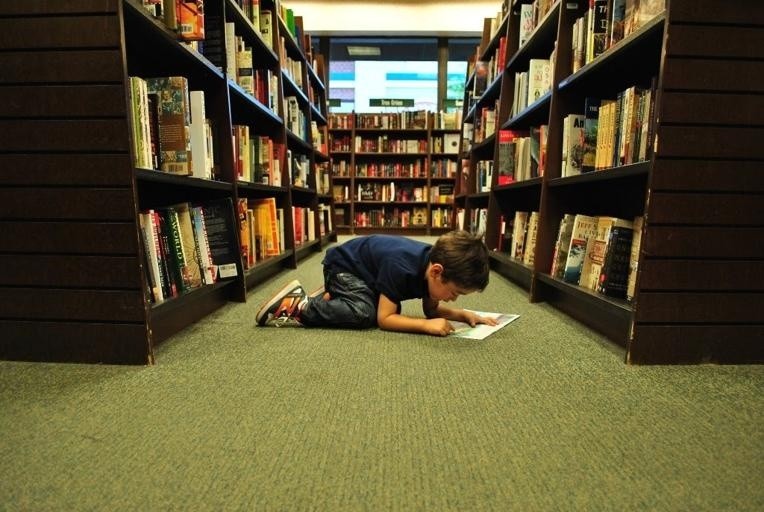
[140,0,326,152]
[461,1,666,298]
[326,110,459,229]
[129,74,331,306]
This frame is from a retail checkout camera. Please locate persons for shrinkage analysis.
[251,230,498,335]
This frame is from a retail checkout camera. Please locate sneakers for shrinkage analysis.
[254,279,307,328]
[294,283,333,324]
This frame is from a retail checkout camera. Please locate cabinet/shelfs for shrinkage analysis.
[458,0,763,367]
[0,1,335,368]
[330,110,459,235]
